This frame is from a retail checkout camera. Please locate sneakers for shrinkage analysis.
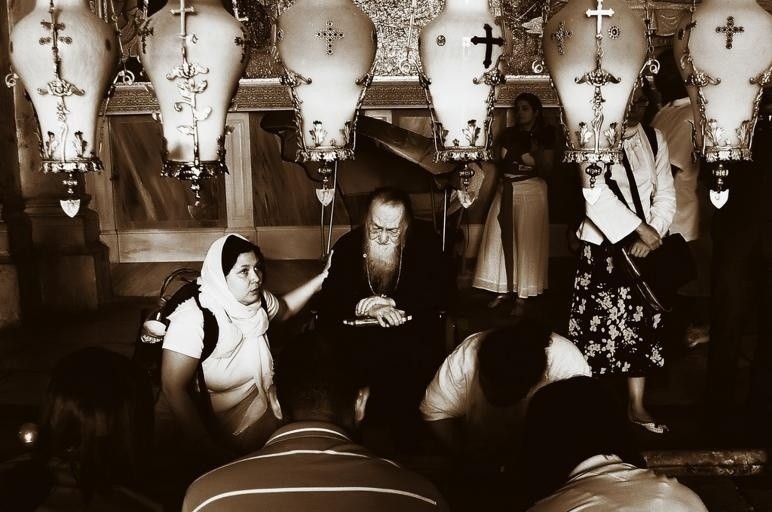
[511,299,524,316]
[488,295,507,308]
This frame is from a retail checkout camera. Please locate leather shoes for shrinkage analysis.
[627,415,670,435]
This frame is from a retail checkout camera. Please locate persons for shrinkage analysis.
[183,344,446,511]
[0,346,180,510]
[566,82,677,437]
[471,92,549,315]
[161,233,335,455]
[649,52,699,263]
[420,309,593,448]
[512,375,712,512]
[323,194,455,421]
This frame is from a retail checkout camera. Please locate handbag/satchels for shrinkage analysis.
[621,233,699,314]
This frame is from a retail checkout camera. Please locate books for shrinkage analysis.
[341,315,413,327]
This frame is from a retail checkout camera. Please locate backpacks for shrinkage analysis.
[136,279,218,381]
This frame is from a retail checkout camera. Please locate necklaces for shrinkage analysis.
[362,244,403,299]
[622,133,639,140]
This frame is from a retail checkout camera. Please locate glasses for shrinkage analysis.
[632,96,649,107]
